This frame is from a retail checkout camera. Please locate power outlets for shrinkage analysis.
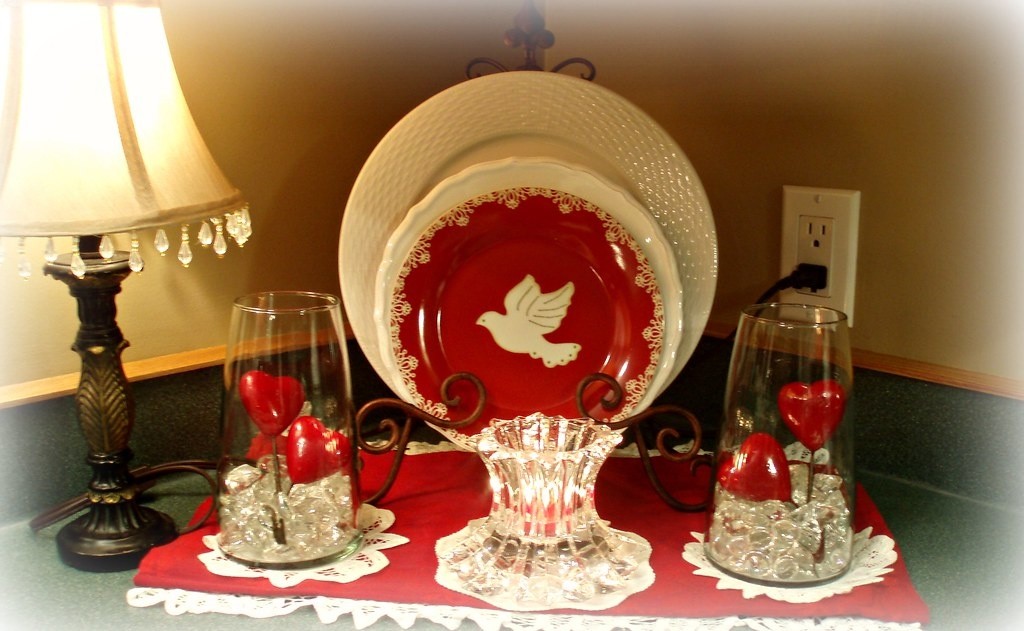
[778,184,860,328]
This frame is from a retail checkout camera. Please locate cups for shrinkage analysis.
[703,302,856,588]
[216,289,364,571]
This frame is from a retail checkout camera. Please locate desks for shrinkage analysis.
[8,418,1024,618]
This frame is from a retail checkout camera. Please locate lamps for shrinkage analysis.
[0,0,251,573]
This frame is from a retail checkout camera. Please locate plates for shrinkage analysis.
[372,157,684,451]
[337,71,719,403]
[388,185,666,450]
